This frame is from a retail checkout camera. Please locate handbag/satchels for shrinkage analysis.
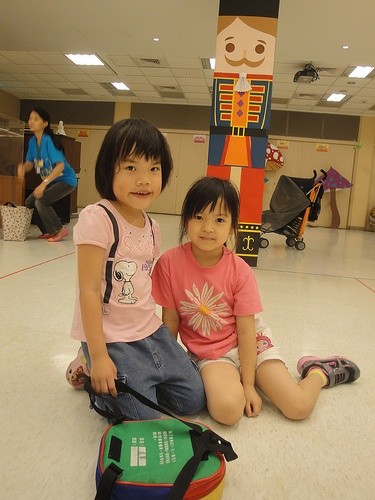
[1,202,35,242]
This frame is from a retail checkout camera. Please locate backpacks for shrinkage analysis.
[84,374,238,500]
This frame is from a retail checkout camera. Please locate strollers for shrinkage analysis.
[259,168,329,252]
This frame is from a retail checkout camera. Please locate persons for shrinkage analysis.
[17,107,77,242]
[65,118,206,421]
[152,176,361,426]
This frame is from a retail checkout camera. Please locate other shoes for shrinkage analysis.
[39,232,55,238]
[49,227,69,242]
[67,347,90,390]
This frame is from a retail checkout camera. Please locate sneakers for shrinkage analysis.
[297,356,361,389]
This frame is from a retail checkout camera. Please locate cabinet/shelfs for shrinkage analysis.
[0,129,24,229]
[23,133,82,217]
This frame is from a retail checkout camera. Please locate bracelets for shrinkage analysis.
[44,178,49,184]
